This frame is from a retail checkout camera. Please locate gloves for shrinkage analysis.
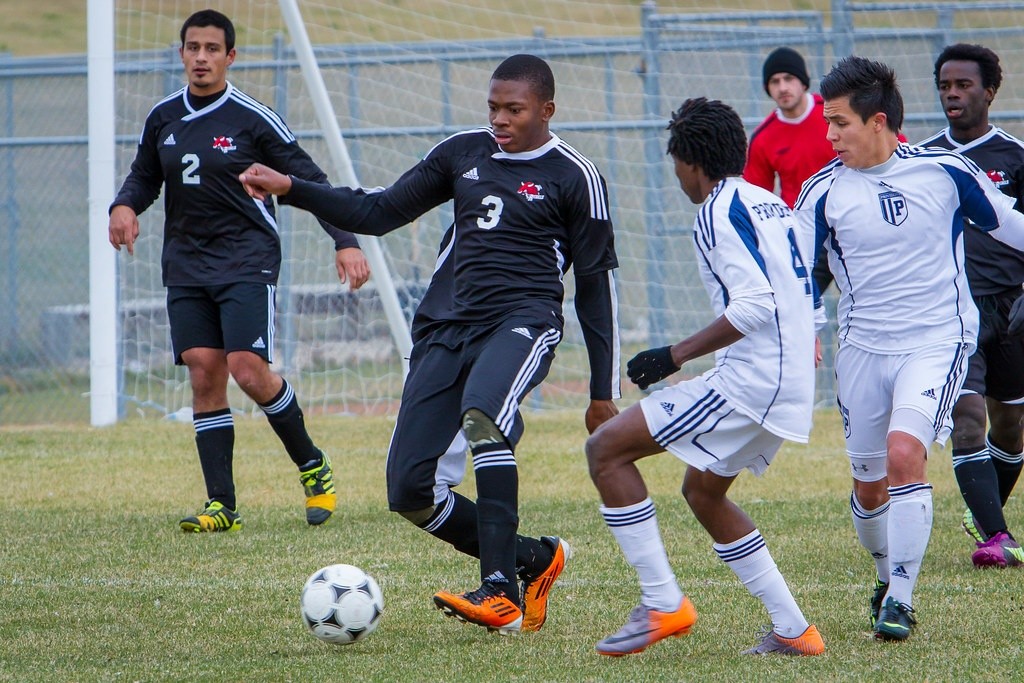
[627,345,681,390]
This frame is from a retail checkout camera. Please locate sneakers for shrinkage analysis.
[597,595,697,658]
[962,507,1023,569]
[179,497,242,533]
[520,536,571,631]
[298,449,336,526]
[871,575,918,642]
[434,581,523,637]
[741,625,825,656]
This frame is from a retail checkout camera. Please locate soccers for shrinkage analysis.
[299,563,383,647]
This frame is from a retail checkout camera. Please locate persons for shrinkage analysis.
[239,53,622,637]
[741,45,907,210]
[107,8,370,531]
[912,42,1024,569]
[792,55,1024,642]
[584,97,825,659]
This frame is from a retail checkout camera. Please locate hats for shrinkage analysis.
[764,48,810,97]
